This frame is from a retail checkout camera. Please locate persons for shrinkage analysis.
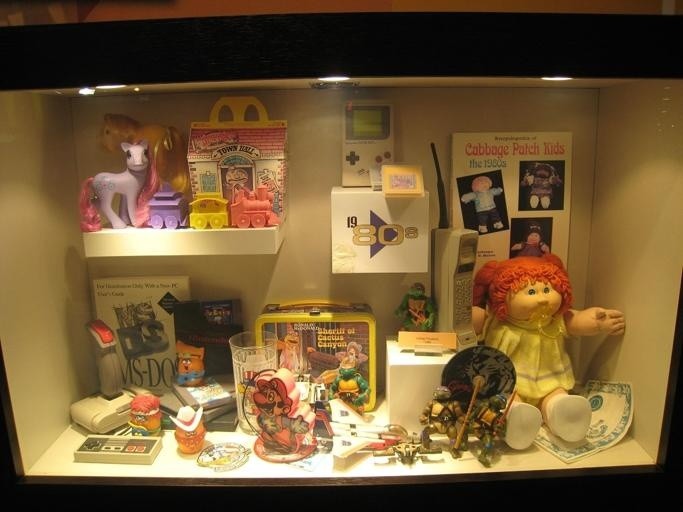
[396,283,435,332]
[461,388,517,466]
[176,338,204,386]
[470,255,625,451]
[326,368,370,416]
[419,381,483,459]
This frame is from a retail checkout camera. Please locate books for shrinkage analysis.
[159,410,238,432]
[171,374,233,410]
[157,392,237,423]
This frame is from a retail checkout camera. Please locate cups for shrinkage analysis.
[227,331,278,434]
